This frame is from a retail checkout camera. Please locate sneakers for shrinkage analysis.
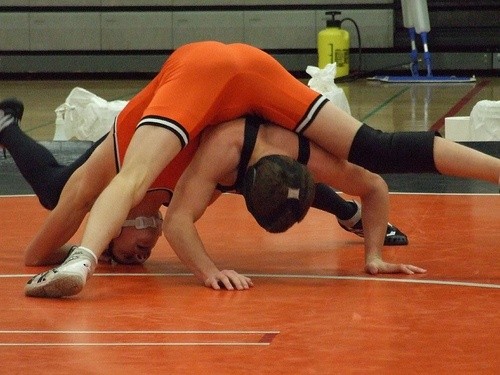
[1,97,24,149]
[22,244,91,298]
[336,199,409,246]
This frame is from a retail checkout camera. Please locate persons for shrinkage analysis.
[23,41,500,298]
[0,97,428,289]
